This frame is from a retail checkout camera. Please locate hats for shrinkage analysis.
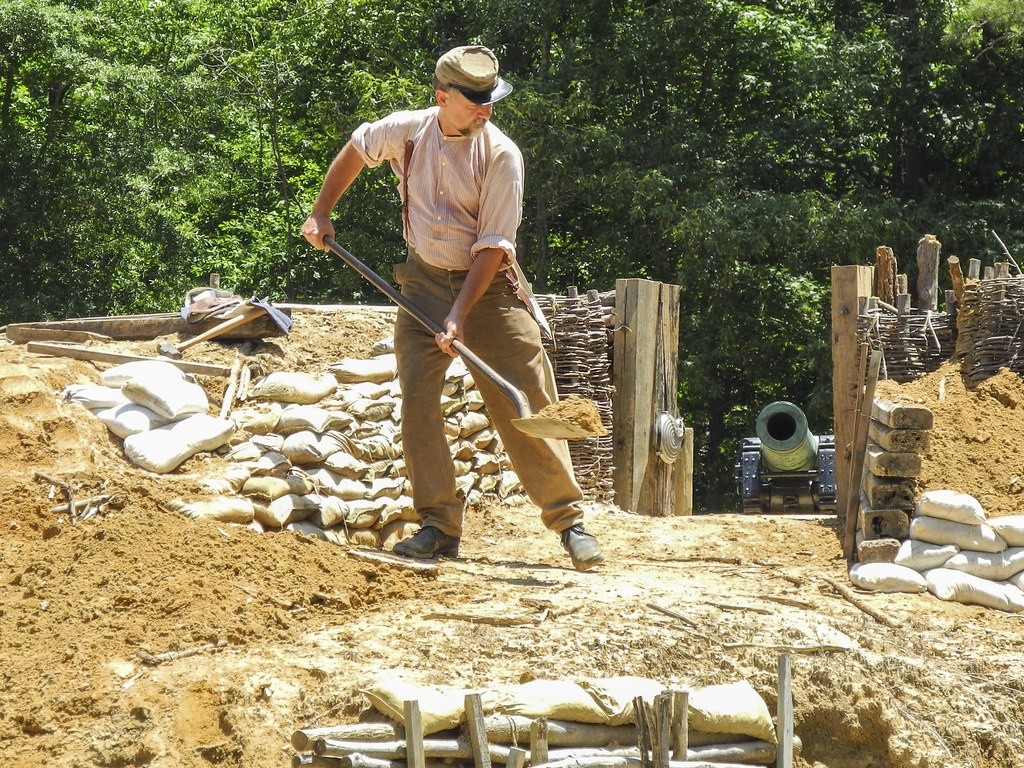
[435,45,513,105]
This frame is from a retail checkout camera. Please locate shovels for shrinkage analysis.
[296,223,609,441]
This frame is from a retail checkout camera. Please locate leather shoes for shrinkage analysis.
[392,526,460,558]
[559,523,605,571]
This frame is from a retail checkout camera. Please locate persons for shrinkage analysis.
[300,46,605,572]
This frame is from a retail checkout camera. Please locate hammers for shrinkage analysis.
[157,307,269,360]
